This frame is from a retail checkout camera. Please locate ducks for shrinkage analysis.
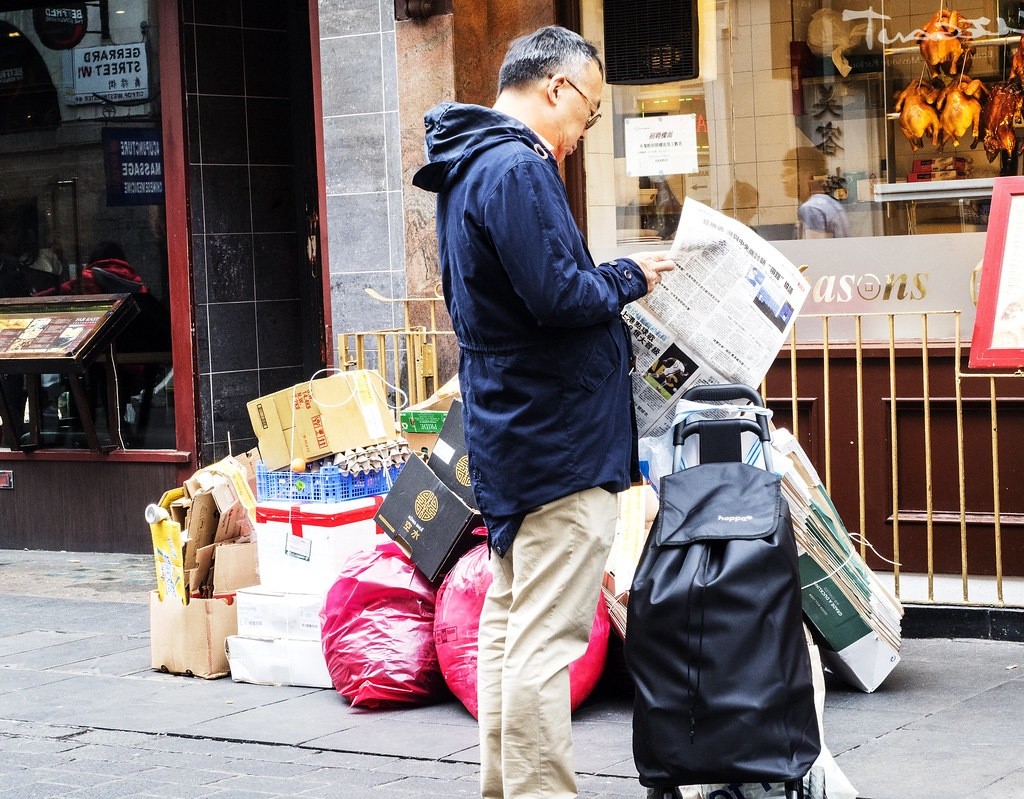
[894,10,1023,163]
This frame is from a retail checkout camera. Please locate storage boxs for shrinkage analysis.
[148,367,901,697]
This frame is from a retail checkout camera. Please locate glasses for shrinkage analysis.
[548,71,602,129]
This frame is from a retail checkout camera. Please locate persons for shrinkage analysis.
[32,242,149,448]
[782,147,850,240]
[0,198,70,450]
[410,26,676,799]
[722,180,760,232]
